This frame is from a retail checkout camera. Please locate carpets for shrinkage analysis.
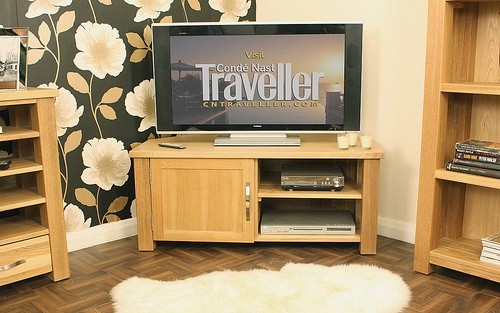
[111,262,413,313]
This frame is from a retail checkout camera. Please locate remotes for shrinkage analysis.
[157,143,186,148]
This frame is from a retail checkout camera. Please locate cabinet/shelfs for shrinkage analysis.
[130,131,384,255]
[0,88,72,289]
[413,0,500,285]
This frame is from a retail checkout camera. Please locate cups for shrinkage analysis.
[347,132,358,146]
[336,133,349,150]
[359,132,374,149]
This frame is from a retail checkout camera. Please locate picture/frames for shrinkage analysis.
[0,26,29,91]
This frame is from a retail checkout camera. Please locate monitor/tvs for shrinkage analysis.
[151,22,363,144]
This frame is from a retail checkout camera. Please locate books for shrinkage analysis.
[446,138,500,179]
[480,231,500,265]
